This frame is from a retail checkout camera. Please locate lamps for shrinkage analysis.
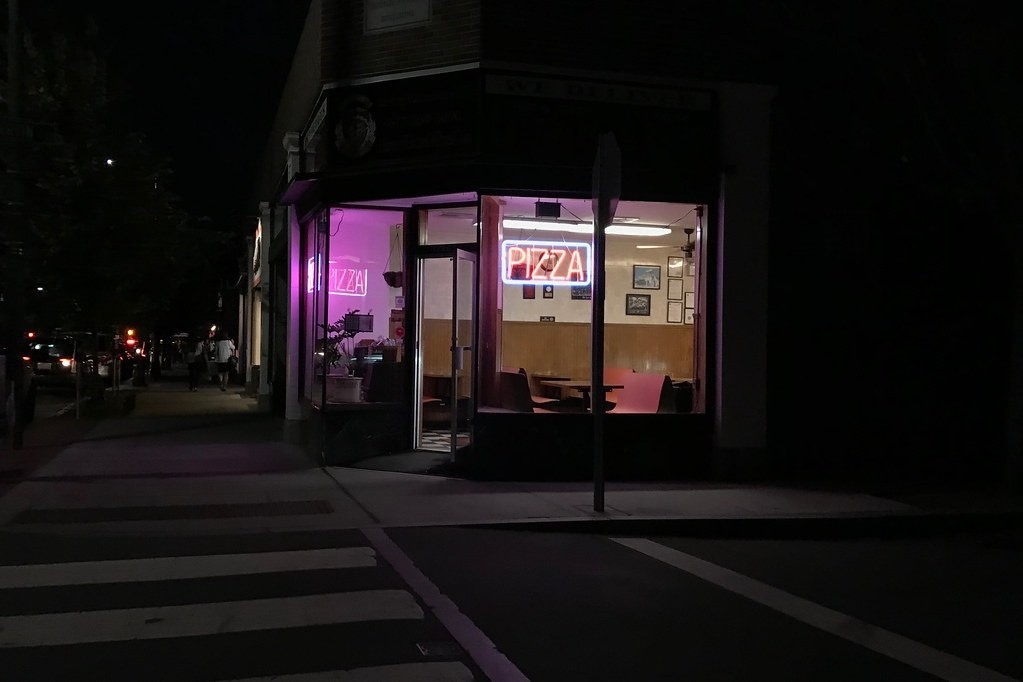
[502,216,671,236]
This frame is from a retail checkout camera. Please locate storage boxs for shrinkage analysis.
[354,347,374,360]
[375,345,401,362]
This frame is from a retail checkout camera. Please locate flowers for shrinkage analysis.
[315,310,363,377]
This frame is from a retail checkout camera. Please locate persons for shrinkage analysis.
[184,330,236,393]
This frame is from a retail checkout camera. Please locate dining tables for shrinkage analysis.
[423,371,466,435]
[541,382,625,413]
[531,373,571,399]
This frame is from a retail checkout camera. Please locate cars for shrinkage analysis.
[31,337,89,398]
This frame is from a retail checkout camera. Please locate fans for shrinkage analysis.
[673,228,695,252]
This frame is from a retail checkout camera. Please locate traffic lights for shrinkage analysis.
[126,327,138,347]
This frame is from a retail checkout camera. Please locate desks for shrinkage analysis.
[350,362,409,403]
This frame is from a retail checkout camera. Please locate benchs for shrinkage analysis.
[611,373,673,413]
[500,371,558,413]
[501,366,561,407]
[603,369,635,403]
[422,394,442,432]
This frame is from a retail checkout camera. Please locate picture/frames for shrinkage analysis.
[523,284,535,299]
[543,285,553,298]
[571,272,591,299]
[626,294,650,316]
[634,265,660,290]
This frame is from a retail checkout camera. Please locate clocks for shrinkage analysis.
[539,251,558,271]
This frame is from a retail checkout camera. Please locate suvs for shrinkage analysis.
[61,332,118,388]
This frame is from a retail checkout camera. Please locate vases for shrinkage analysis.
[337,377,364,403]
